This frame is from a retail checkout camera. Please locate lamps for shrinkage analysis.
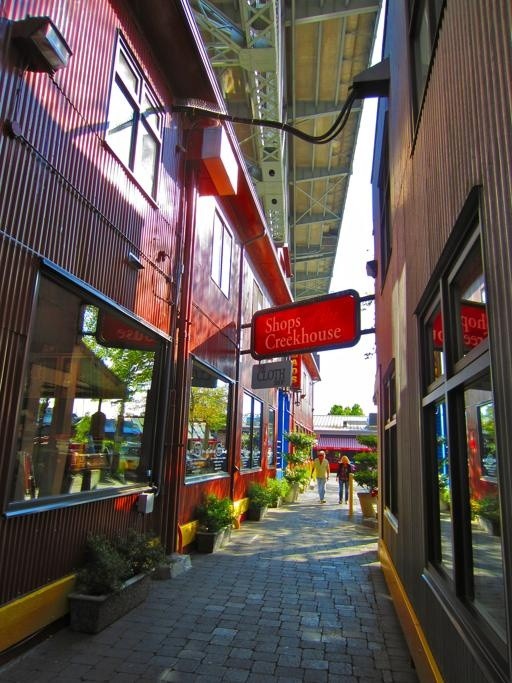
[11,14,73,72]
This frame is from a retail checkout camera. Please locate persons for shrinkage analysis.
[310,449,331,503]
[335,455,352,504]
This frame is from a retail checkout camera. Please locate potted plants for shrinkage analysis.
[353,434,378,518]
[248,432,318,521]
[473,498,500,535]
[67,528,178,634]
[193,495,237,553]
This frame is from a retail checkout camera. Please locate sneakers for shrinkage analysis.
[320,499,348,505]
